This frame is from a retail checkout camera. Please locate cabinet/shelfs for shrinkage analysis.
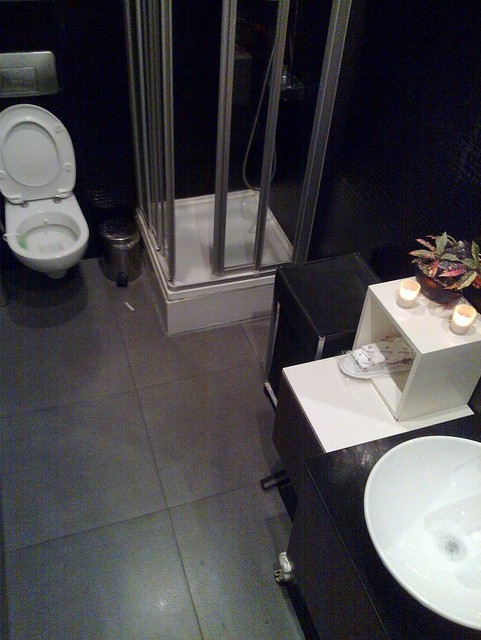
[282,459,386,640]
[350,275,480,423]
[262,252,381,411]
[273,351,474,496]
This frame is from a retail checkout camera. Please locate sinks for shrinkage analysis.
[362,434,481,632]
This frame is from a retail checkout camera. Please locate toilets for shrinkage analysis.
[0,103,89,279]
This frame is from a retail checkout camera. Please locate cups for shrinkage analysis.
[448,301,476,336]
[395,277,422,311]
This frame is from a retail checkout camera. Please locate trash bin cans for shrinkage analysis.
[98,215,142,286]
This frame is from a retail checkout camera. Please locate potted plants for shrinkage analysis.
[409,232,481,305]
